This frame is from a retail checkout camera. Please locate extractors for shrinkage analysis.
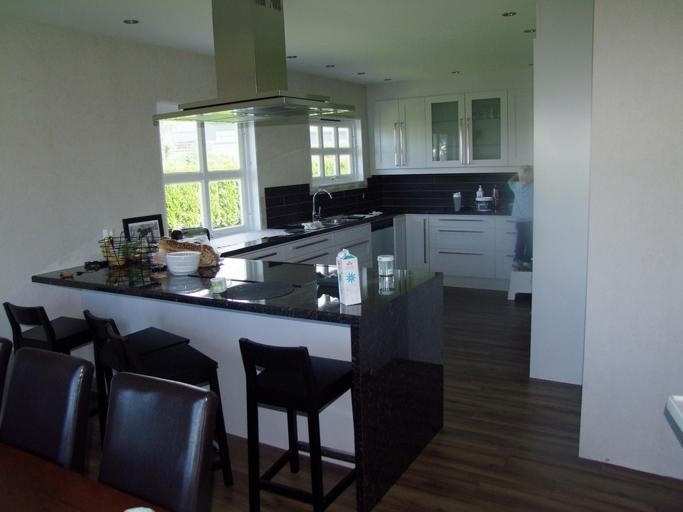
[151,0,354,127]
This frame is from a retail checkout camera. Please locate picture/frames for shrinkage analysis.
[122,214,168,272]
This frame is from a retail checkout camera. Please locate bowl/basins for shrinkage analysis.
[166,251,201,276]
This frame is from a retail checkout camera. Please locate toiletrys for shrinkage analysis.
[476,184,484,198]
[492,185,499,200]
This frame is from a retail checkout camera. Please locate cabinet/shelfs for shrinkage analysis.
[227,212,518,292]
[371,88,534,172]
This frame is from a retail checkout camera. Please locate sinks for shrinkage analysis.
[321,216,372,225]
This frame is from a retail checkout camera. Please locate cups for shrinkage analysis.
[378,277,395,296]
[377,254,394,276]
[209,277,227,294]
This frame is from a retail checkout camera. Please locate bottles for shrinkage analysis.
[452,192,461,211]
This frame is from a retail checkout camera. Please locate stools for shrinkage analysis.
[507,270,532,302]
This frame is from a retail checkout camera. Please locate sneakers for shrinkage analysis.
[511,259,532,270]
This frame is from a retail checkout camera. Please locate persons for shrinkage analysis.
[508,165,533,271]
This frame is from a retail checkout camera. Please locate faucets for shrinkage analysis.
[313,189,333,211]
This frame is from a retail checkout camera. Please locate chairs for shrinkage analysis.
[0,346,94,478]
[0,336,13,390]
[96,366,219,512]
[3,303,93,357]
[92,325,233,487]
[81,301,192,368]
[239,338,356,512]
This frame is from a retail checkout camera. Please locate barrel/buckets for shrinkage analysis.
[476,197,494,211]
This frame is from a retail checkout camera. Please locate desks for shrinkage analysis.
[0,442,169,512]
